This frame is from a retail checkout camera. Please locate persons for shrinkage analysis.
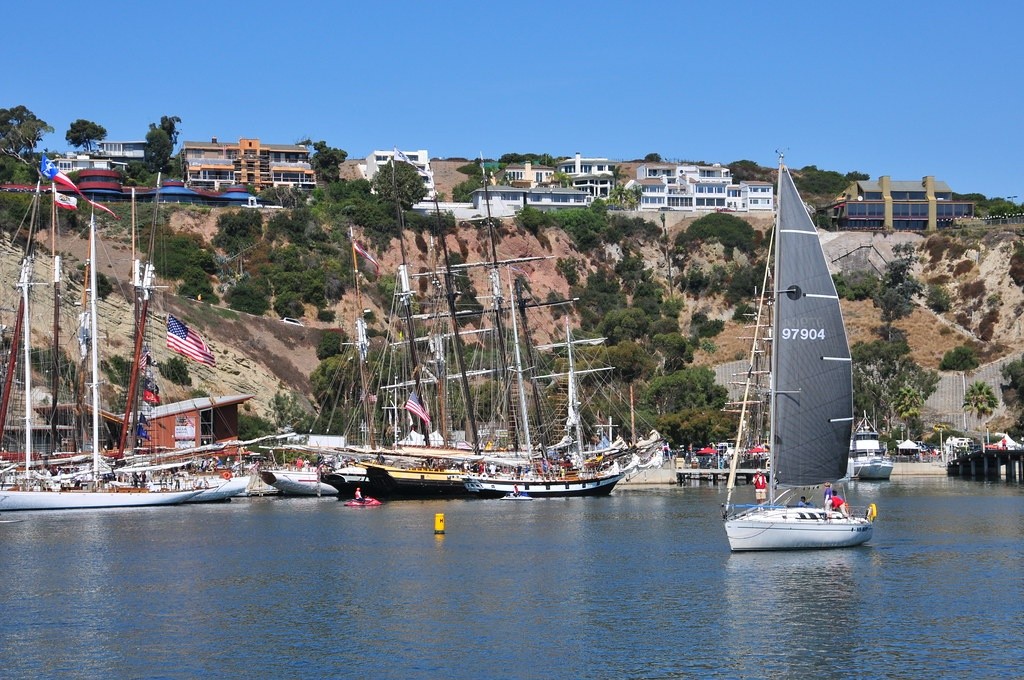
[297,454,309,471]
[471,459,552,478]
[799,481,847,518]
[355,488,365,501]
[753,469,766,511]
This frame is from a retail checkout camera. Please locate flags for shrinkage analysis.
[138,353,160,440]
[405,391,430,425]
[167,314,216,368]
[55,191,77,210]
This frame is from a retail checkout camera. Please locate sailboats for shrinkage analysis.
[1,152,679,515]
[720,145,879,554]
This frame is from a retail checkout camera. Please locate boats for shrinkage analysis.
[847,404,894,480]
[343,494,382,507]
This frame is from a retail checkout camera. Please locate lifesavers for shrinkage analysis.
[481,473,488,480]
[868,503,877,521]
[422,461,428,468]
[223,472,230,479]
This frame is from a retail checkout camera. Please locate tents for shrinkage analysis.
[897,439,920,455]
[398,430,443,446]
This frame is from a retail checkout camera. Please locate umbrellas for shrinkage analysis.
[748,445,769,453]
[696,447,717,453]
